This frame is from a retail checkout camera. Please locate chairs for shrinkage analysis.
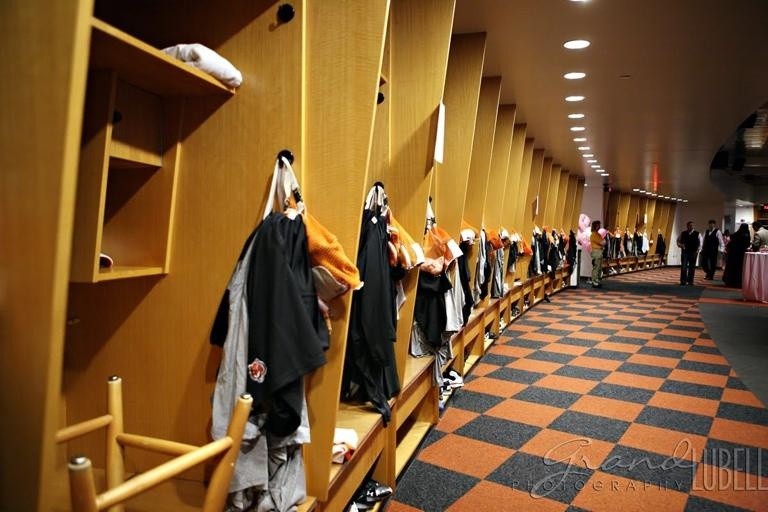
[353,475,393,503]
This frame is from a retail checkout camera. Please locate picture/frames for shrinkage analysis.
[742,252,768,303]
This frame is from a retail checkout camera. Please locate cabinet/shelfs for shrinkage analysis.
[0,0,675,512]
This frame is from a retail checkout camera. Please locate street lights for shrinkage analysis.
[270,156,365,302]
[420,199,464,277]
[487,228,505,251]
[380,187,426,270]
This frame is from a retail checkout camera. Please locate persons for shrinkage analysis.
[722,229,731,271]
[677,221,703,287]
[752,221,768,252]
[722,224,750,288]
[701,220,725,280]
[590,220,606,288]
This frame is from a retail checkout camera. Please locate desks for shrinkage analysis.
[742,252,768,303]
